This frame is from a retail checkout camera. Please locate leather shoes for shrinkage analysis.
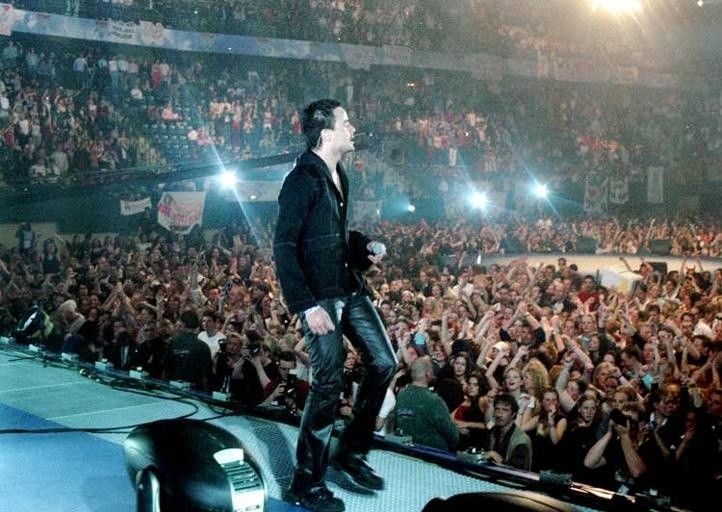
[329,448,383,490]
[284,470,345,511]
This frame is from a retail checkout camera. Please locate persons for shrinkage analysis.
[2,212,721,512]
[270,98,397,510]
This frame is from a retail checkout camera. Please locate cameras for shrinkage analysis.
[579,333,591,352]
[637,371,655,389]
[609,410,635,431]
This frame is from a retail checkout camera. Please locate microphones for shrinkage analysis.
[362,243,387,270]
